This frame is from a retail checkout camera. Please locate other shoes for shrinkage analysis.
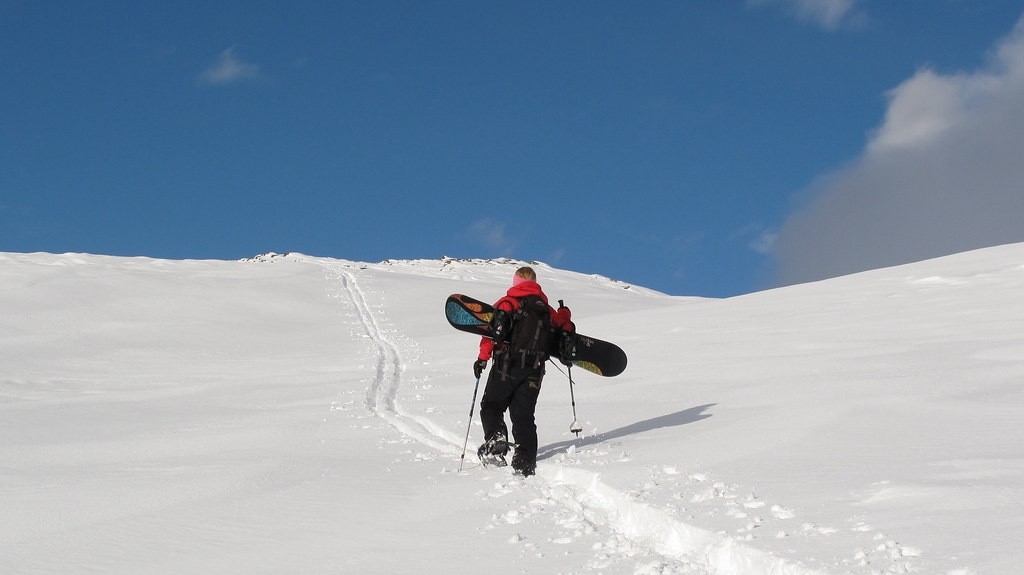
[510,456,537,477]
[478,433,511,460]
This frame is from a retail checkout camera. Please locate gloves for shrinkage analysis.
[473,358,486,379]
[556,306,572,320]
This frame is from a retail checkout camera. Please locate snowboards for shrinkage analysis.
[444,293,628,378]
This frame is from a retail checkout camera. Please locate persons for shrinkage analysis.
[470,267,574,477]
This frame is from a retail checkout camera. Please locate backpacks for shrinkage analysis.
[510,295,552,362]
